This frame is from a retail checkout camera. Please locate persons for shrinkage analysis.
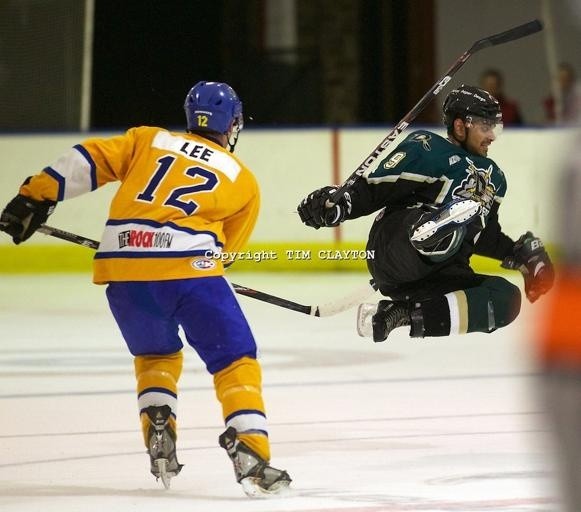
[1,79,297,494]
[543,60,580,128]
[296,82,558,345]
[479,64,525,131]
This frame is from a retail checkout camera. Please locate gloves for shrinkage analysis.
[297,185,353,230]
[501,231,555,303]
[1,193,56,245]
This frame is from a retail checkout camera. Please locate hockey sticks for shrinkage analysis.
[32,225,376,316]
[325,20,544,207]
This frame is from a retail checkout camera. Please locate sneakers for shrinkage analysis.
[219,426,292,492]
[372,299,411,343]
[408,197,483,249]
[146,405,183,479]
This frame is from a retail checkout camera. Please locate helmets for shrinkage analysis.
[442,85,504,147]
[183,81,244,134]
